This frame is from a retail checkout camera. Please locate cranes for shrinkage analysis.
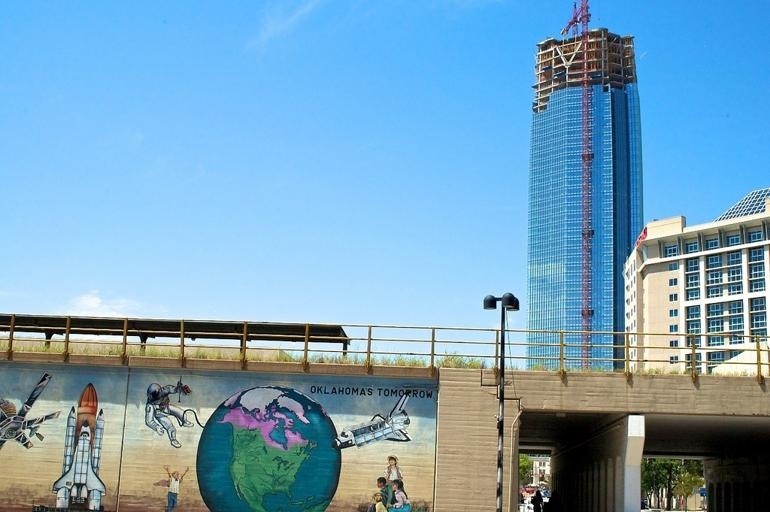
[560,1,591,370]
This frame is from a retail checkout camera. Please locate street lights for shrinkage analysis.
[484,293,519,511]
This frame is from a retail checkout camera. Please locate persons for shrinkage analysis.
[162,465,189,511]
[145,383,195,448]
[642,498,648,509]
[369,454,412,512]
[531,490,544,512]
